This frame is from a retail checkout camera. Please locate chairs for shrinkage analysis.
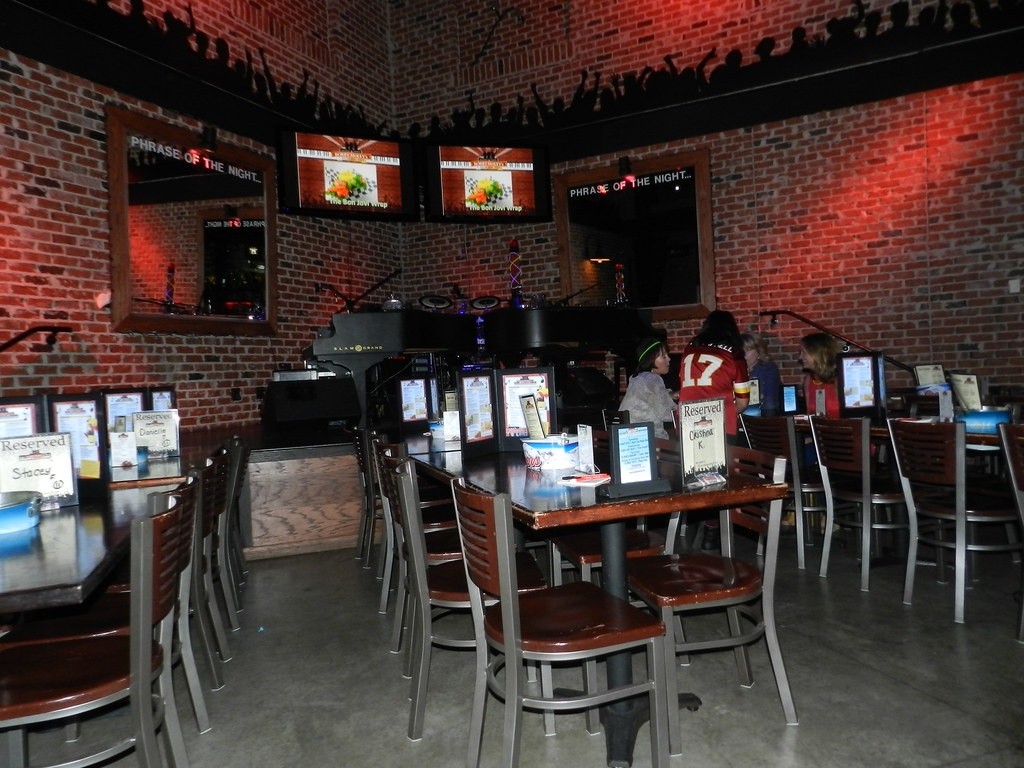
[0,428,251,768]
[352,373,1024,768]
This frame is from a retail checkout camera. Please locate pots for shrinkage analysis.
[0,490,43,536]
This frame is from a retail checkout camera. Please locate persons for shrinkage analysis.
[739,330,795,527]
[673,306,751,554]
[612,333,679,460]
[799,331,877,535]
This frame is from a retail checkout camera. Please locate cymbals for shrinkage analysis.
[471,295,499,309]
[419,295,451,309]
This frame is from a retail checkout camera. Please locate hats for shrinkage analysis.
[634,338,660,361]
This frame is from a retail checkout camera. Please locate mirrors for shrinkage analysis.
[104,103,279,337]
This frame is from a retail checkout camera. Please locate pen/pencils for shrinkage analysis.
[562,473,608,480]
[576,476,610,481]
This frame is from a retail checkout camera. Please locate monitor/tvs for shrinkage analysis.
[273,122,420,223]
[420,133,553,224]
